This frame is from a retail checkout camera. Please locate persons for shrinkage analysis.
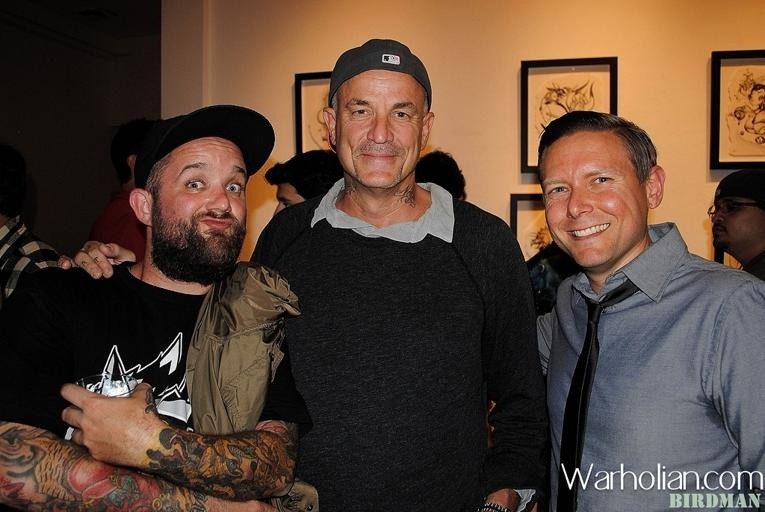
[531,111,765,511]
[709,168,761,279]
[266,150,343,219]
[61,38,545,510]
[415,150,466,202]
[98,121,157,262]
[4,146,61,314]
[1,104,311,510]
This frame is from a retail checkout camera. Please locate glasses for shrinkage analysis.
[707,200,760,218]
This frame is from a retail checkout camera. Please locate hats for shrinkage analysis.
[132,103,276,191]
[712,167,765,203]
[328,38,432,113]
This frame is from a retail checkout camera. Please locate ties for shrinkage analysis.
[555,279,639,511]
